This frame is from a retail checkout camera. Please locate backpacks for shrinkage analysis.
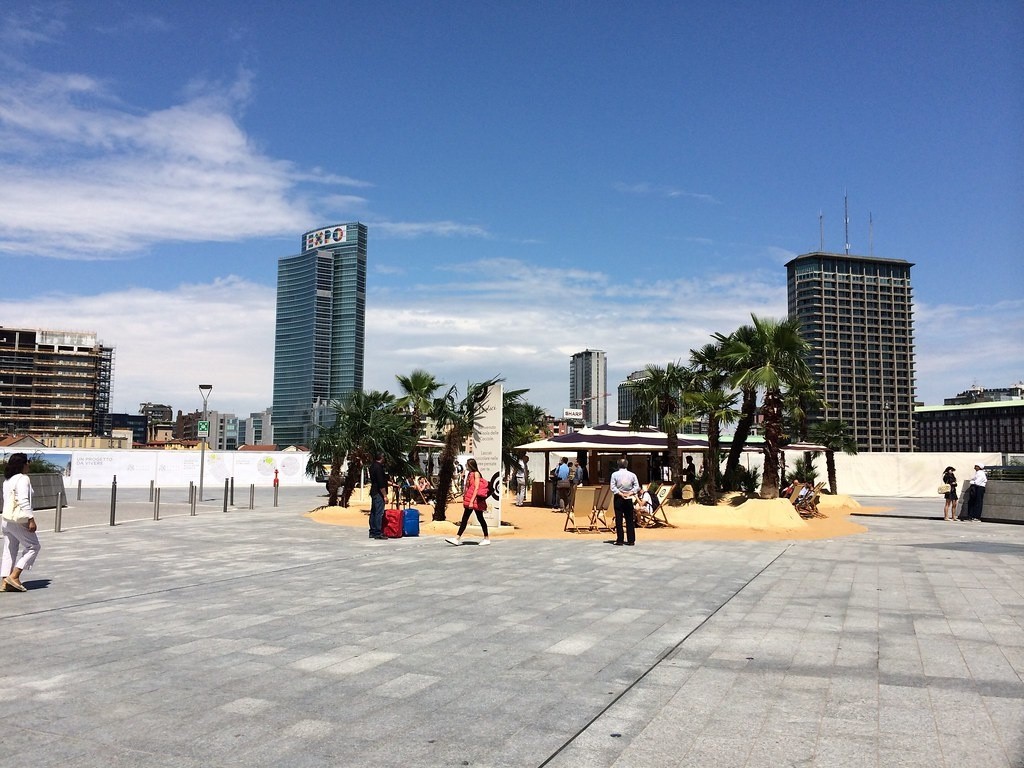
[642,491,659,513]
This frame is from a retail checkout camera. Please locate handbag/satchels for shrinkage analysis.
[477,472,494,500]
[938,483,951,494]
[2,487,30,523]
[556,479,571,489]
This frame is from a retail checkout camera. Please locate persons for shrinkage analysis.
[404,452,466,507]
[629,483,655,525]
[545,452,585,512]
[1,453,40,595]
[515,454,532,508]
[367,451,391,538]
[681,454,696,495]
[942,466,961,522]
[966,464,989,525]
[780,477,822,518]
[611,457,641,544]
[446,457,491,545]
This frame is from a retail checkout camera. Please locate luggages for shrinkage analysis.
[381,484,404,537]
[402,484,419,537]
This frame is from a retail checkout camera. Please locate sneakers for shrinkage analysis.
[445,538,459,546]
[478,539,490,546]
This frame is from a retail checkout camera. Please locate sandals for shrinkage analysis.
[0,586,18,592]
[4,576,27,591]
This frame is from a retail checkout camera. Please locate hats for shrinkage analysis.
[974,463,984,470]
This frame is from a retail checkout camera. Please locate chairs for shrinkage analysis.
[637,483,677,530]
[811,482,829,517]
[796,481,822,518]
[782,483,805,507]
[562,483,600,534]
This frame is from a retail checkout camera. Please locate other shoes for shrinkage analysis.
[375,535,388,539]
[613,541,623,545]
[624,542,634,545]
[369,534,383,538]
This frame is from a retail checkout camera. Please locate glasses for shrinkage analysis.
[27,460,30,464]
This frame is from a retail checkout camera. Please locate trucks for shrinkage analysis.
[314,443,372,493]
